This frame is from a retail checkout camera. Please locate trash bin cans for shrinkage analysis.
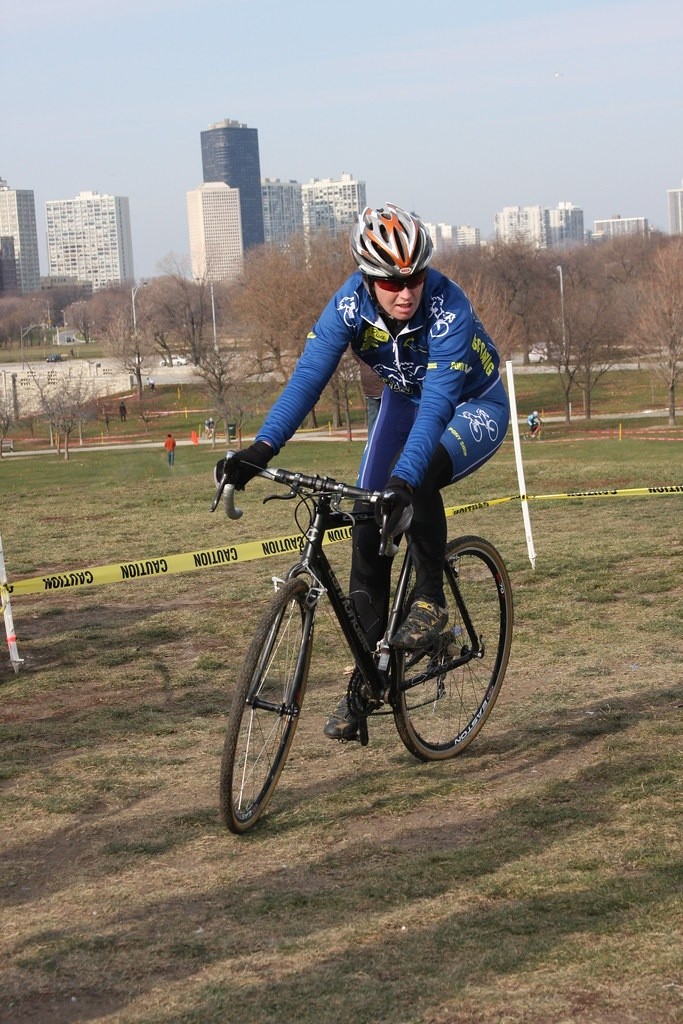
[227,423,237,439]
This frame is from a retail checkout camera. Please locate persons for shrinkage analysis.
[216,204,509,739]
[524,411,542,438]
[205,417,214,439]
[120,402,126,422]
[147,378,154,391]
[165,434,176,466]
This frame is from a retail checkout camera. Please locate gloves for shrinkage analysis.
[376,478,414,538]
[212,442,276,491]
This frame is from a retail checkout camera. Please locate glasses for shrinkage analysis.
[370,266,430,293]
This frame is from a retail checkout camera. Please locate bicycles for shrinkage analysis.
[523,421,546,441]
[211,450,514,836]
[148,383,158,393]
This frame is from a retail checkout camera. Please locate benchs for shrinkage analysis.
[2,440,14,453]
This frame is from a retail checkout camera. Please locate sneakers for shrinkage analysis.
[393,593,450,648]
[324,693,374,739]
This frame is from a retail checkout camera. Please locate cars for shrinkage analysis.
[46,352,65,362]
[66,337,71,342]
[159,354,188,366]
[519,342,554,363]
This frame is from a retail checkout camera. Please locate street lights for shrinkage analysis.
[131,281,149,391]
[192,276,218,354]
[556,265,567,363]
[20,323,47,370]
[60,310,66,331]
[32,297,51,330]
[55,326,59,345]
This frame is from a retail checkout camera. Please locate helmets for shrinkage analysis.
[349,202,432,279]
